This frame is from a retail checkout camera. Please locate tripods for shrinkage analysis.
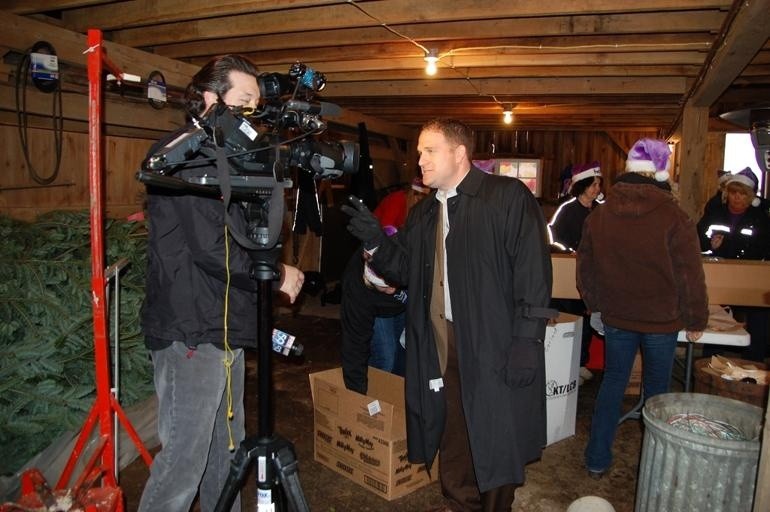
[137,171,310,511]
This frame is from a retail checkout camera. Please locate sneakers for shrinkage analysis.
[578,365,594,386]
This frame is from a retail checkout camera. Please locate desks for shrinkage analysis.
[617,301,751,426]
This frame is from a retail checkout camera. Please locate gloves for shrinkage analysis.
[338,192,388,252]
[492,334,546,391]
[588,311,604,334]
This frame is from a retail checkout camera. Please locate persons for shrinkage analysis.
[340,115,561,509]
[567,136,710,478]
[699,166,770,360]
[703,169,736,215]
[129,52,307,509]
[337,177,440,394]
[545,158,606,382]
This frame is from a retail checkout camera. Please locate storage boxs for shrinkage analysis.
[308,363,440,503]
[541,311,584,448]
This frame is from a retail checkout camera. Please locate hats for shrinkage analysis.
[560,161,604,198]
[362,223,401,288]
[623,136,673,184]
[714,164,759,195]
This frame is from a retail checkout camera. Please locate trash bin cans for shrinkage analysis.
[696,357,768,407]
[635,392,763,512]
